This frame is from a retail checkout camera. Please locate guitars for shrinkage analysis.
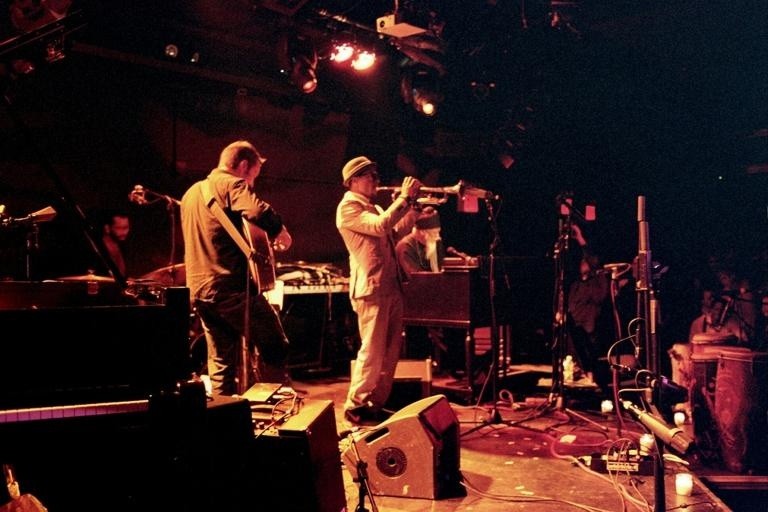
[242,217,276,295]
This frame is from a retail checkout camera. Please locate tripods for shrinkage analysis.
[509,217,607,432]
[458,206,557,439]
[473,253,614,421]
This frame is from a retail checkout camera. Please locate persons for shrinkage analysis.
[180,141,308,401]
[335,155,432,422]
[690,268,768,345]
[395,206,467,378]
[74,213,129,297]
[568,253,609,373]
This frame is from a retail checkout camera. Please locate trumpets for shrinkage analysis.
[376,180,465,206]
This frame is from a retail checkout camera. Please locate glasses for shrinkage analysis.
[356,172,379,180]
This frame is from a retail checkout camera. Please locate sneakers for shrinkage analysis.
[346,408,383,426]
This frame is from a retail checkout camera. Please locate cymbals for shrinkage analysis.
[141,263,186,287]
[56,274,116,283]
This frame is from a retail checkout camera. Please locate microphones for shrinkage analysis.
[647,370,687,400]
[134,184,150,193]
[610,364,647,381]
[603,262,628,271]
[618,397,695,458]
[447,246,471,261]
[466,188,499,201]
[556,194,585,219]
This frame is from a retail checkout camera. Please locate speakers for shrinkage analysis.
[350,358,432,414]
[344,393,461,501]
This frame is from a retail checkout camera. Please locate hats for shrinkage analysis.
[415,207,440,229]
[341,156,377,186]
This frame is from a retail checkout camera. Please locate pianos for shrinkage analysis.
[401,257,554,403]
[1,285,259,512]
